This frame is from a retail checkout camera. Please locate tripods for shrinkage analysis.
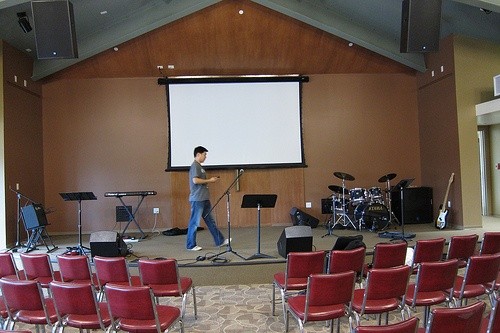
[59,192,98,258]
[389,178,416,244]
[321,177,358,240]
[204,171,246,260]
[240,194,277,260]
[5,187,40,252]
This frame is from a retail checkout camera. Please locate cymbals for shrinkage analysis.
[328,186,349,194]
[378,173,397,183]
[332,172,355,181]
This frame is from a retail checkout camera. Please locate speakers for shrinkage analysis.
[89,231,128,262]
[400,0,442,54]
[290,207,319,228]
[390,186,434,224]
[21,204,48,229]
[31,0,79,60]
[277,225,313,258]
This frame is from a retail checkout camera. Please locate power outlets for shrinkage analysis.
[153,208,159,213]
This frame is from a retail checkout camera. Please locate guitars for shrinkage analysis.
[435,172,455,230]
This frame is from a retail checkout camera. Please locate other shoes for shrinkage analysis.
[187,245,202,250]
[220,237,232,247]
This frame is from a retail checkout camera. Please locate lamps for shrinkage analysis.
[16,12,32,34]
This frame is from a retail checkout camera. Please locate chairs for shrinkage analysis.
[0,253,198,333]
[272,232,500,333]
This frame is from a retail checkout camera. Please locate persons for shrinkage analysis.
[186,146,232,250]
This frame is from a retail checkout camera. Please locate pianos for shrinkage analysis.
[104,190,157,240]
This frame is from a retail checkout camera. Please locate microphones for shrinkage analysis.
[240,169,244,172]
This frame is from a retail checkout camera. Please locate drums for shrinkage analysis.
[330,199,348,213]
[349,188,366,202]
[368,187,382,198]
[354,202,390,230]
